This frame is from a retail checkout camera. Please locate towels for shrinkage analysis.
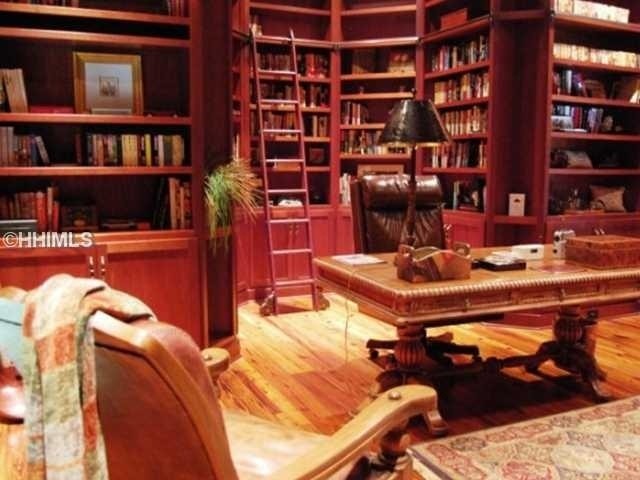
[17,273,158,480]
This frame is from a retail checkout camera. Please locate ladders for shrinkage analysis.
[250,23,330,316]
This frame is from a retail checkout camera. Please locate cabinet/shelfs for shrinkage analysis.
[0,0,239,361]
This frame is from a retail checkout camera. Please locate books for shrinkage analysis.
[331,252,387,267]
[0,67,194,237]
[230,0,640,217]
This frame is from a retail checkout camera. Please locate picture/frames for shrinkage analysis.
[73,50,144,117]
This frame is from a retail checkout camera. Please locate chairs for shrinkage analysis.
[0,282,440,479]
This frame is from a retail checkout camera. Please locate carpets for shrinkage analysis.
[404,392,640,480]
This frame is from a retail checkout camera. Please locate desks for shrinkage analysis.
[313,240,639,431]
